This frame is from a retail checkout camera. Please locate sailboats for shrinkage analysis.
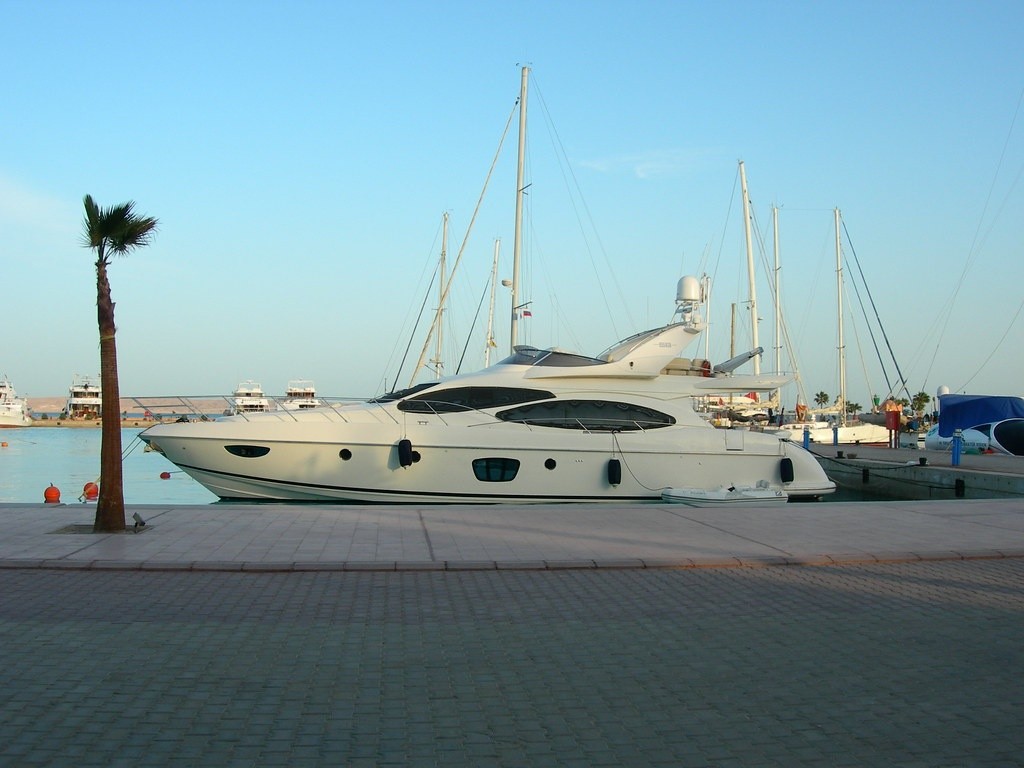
[404,64,928,451]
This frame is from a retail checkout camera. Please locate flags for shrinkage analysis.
[489,340,497,347]
[524,310,533,317]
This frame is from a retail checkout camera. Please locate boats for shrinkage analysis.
[230,381,270,414]
[66,374,102,420]
[276,379,321,412]
[924,385,1024,458]
[135,275,837,504]
[0,376,32,429]
[662,479,788,507]
